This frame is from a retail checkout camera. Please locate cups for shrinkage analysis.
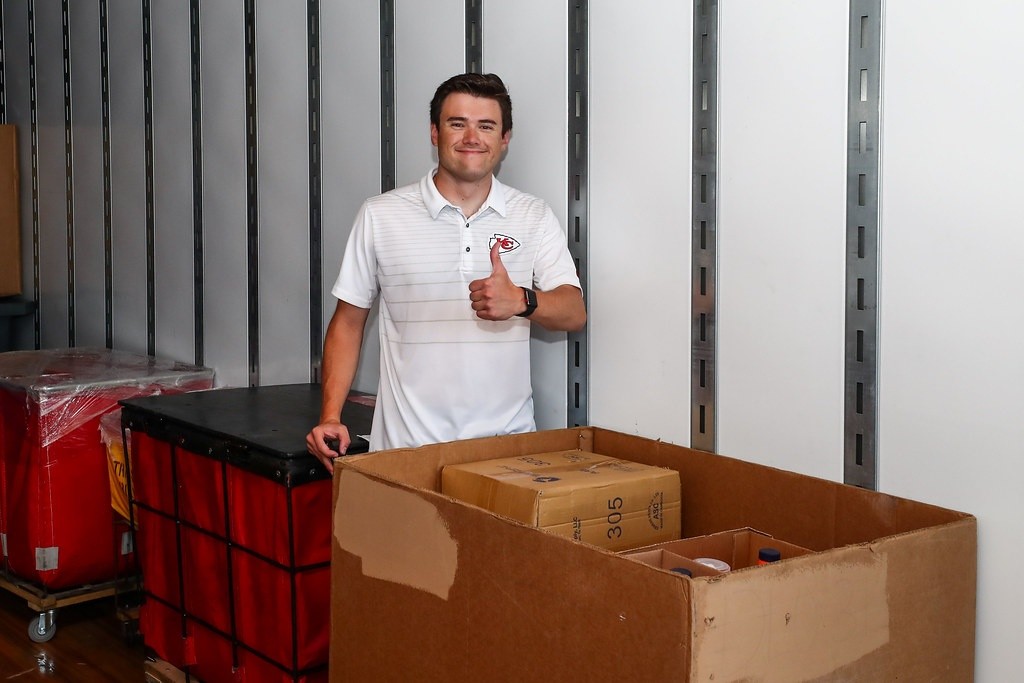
[694,557,731,573]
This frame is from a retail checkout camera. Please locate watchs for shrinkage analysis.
[515,286,537,318]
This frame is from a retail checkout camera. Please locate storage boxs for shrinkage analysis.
[327,424,976,682]
[118,382,377,683]
[0,345,216,590]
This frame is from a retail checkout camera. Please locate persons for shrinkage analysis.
[305,72,586,477]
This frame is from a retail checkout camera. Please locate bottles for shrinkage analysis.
[758,547,780,566]
[670,568,692,578]
